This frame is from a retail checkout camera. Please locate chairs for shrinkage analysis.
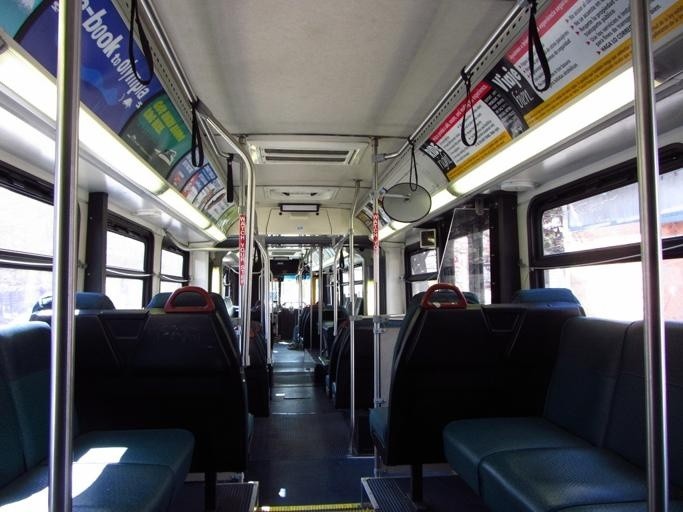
[30,286,248,512]
[300,283,586,500]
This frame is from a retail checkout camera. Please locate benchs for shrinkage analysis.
[442,319,682,512]
[0,320,195,512]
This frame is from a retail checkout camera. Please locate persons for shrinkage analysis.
[287,325,300,348]
[256,299,273,350]
